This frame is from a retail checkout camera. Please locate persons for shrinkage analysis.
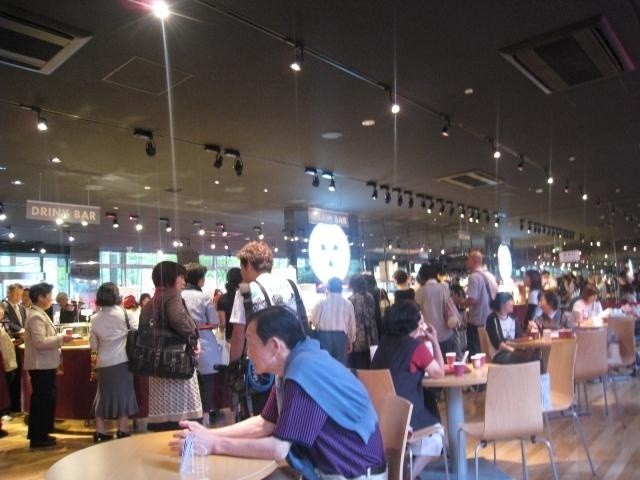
[24,283,73,449]
[571,283,604,328]
[88,298,101,322]
[365,275,390,342]
[0,303,26,439]
[139,293,153,317]
[56,292,77,323]
[480,251,498,295]
[451,250,497,393]
[415,264,455,363]
[525,290,570,335]
[349,274,375,370]
[310,277,356,368]
[20,288,32,309]
[604,256,640,319]
[0,284,28,413]
[138,260,204,430]
[88,282,139,443]
[217,268,249,426]
[180,262,228,426]
[449,284,468,392]
[393,270,415,304]
[122,293,139,328]
[228,240,311,424]
[169,306,389,480]
[372,302,444,480]
[486,292,540,359]
[545,264,604,282]
[212,289,223,311]
[521,270,546,339]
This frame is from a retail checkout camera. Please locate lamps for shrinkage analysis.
[271,245,278,252]
[517,160,524,171]
[288,56,303,69]
[221,228,228,237]
[397,196,403,206]
[580,191,587,200]
[198,227,205,235]
[460,209,466,218]
[134,221,143,231]
[439,205,445,216]
[37,118,47,132]
[234,161,240,174]
[372,190,378,200]
[290,234,294,242]
[67,231,74,241]
[494,217,500,227]
[112,219,118,229]
[258,232,264,239]
[0,206,5,220]
[145,142,156,154]
[390,102,399,113]
[491,146,501,158]
[302,234,307,242]
[440,245,445,254]
[387,240,392,249]
[397,242,401,248]
[439,124,450,136]
[528,226,532,233]
[546,175,554,184]
[520,224,523,230]
[427,203,434,213]
[421,201,426,208]
[295,235,299,242]
[312,175,319,187]
[329,181,335,192]
[448,207,454,215]
[469,212,475,222]
[213,157,223,170]
[408,198,414,208]
[385,193,391,204]
[283,234,289,241]
[165,223,172,233]
[487,216,490,221]
[427,248,431,252]
[476,213,481,223]
[224,242,229,249]
[564,186,568,193]
[8,229,13,238]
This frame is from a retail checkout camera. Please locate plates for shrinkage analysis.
[58,333,82,340]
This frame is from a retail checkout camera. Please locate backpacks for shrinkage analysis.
[214,282,275,396]
[252,278,312,337]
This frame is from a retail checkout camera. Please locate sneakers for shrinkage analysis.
[30,435,56,448]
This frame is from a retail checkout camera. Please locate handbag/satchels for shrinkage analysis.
[380,288,389,319]
[442,282,460,329]
[128,330,195,380]
[123,309,137,357]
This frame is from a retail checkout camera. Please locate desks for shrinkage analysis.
[507,337,588,417]
[421,362,512,479]
[46,430,280,480]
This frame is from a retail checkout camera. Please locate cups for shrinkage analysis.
[531,330,539,340]
[65,329,72,339]
[454,363,464,380]
[471,356,481,369]
[180,440,208,478]
[477,352,486,367]
[446,352,456,367]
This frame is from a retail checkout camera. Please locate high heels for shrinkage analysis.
[93,432,113,443]
[117,431,129,438]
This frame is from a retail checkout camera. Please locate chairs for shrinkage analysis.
[356,370,448,480]
[457,366,557,480]
[584,316,635,417]
[375,392,414,479]
[572,330,627,432]
[478,327,491,364]
[536,339,596,476]
